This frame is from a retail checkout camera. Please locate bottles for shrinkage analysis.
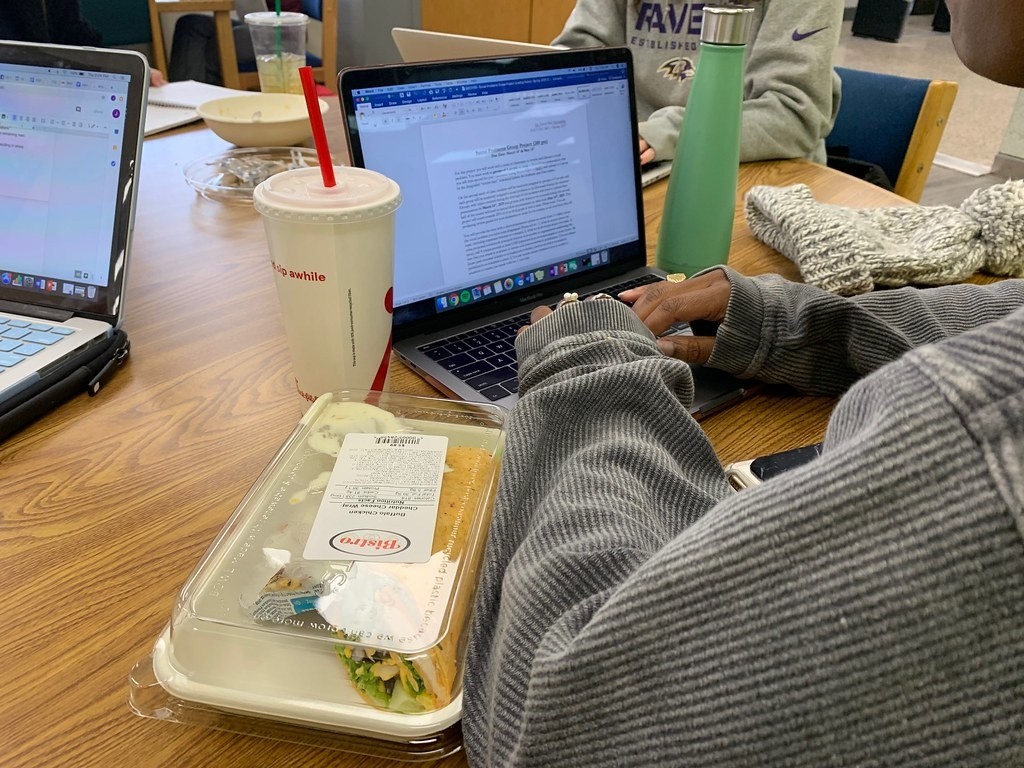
[654,5,755,284]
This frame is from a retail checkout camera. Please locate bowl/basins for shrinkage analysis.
[127,387,510,767]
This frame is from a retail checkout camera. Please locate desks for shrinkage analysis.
[0,78,1023,768]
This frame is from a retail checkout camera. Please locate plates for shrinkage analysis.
[183,146,345,207]
[196,94,329,150]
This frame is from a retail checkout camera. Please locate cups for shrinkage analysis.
[243,10,308,99]
[252,166,401,431]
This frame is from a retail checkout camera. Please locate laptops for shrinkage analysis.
[0,39,151,414]
[391,27,672,189]
[338,47,768,430]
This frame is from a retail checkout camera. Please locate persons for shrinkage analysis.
[154,0,225,92]
[432,0,1024,768]
[545,0,847,177]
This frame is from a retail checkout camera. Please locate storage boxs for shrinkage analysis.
[128,389,509,764]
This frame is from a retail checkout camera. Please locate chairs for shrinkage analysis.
[815,65,957,205]
[235,0,338,94]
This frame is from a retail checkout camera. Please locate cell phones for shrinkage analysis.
[725,441,825,494]
